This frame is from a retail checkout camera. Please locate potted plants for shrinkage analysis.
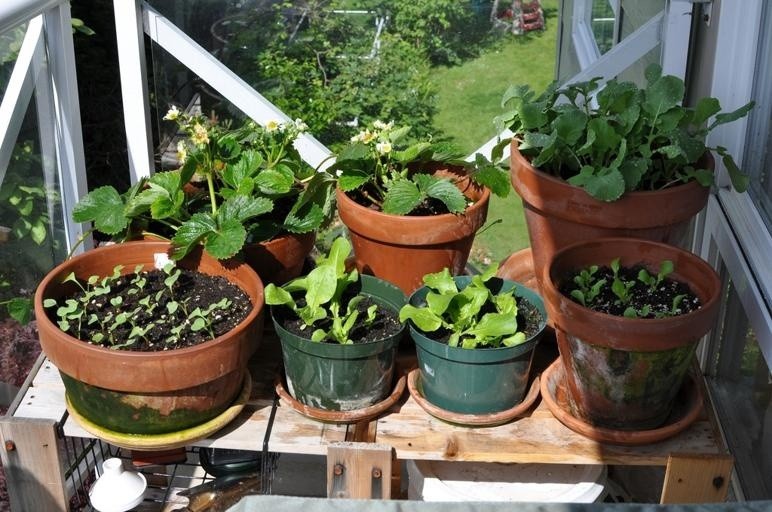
[33,242,264,437]
[493,63,755,326]
[264,236,408,410]
[401,261,549,414]
[543,238,723,432]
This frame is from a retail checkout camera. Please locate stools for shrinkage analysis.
[489,0,546,35]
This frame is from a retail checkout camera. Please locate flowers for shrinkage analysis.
[290,118,511,214]
[5,106,332,326]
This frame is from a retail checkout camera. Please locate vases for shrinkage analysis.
[333,165,491,302]
[127,176,319,333]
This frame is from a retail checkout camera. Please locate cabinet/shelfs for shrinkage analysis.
[0,349,735,512]
